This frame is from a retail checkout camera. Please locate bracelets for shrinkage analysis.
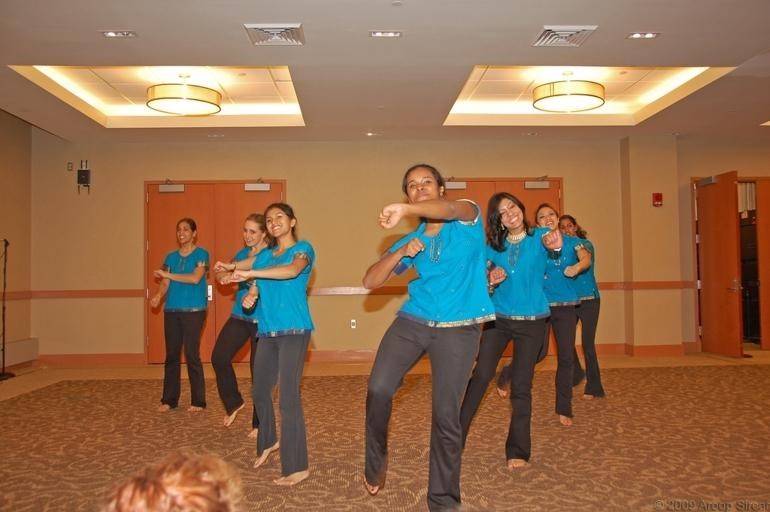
[232,261,237,271]
[487,274,496,290]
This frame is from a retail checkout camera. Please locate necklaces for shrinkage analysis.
[551,251,561,266]
[506,230,527,274]
[429,222,442,263]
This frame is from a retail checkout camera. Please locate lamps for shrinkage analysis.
[143,66,223,120]
[532,69,608,115]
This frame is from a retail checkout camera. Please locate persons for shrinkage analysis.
[149,217,210,413]
[213,201,317,486]
[211,213,272,428]
[362,162,496,512]
[103,445,247,512]
[558,213,605,398]
[498,203,593,428]
[461,191,563,471]
